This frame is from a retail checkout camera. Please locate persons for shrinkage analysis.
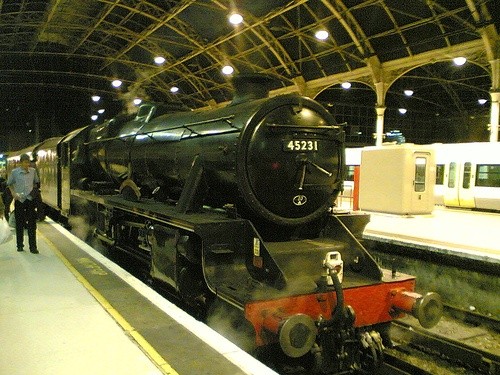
[0,168,13,223]
[7,154,41,254]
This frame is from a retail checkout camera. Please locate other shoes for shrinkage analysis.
[30,249,39,254]
[17,248,23,251]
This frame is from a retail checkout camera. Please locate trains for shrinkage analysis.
[0,92,442,370]
[346,141,500,212]
